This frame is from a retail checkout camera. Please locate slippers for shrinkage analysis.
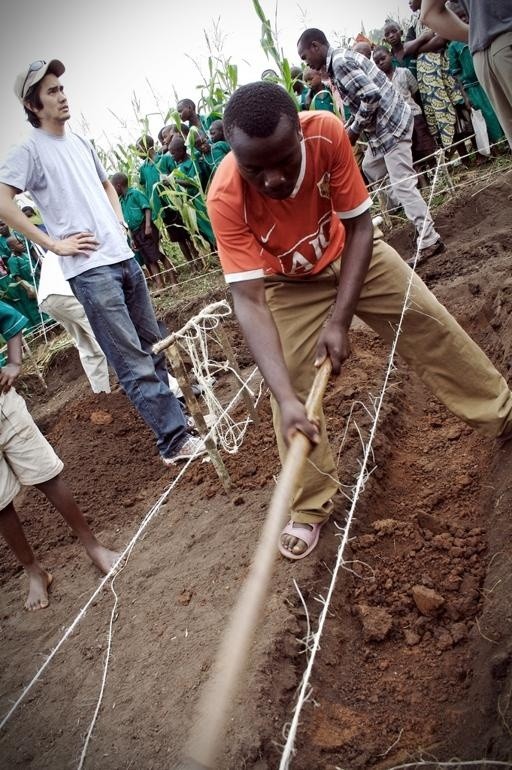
[279,515,329,559]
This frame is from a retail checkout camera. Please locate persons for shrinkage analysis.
[37,251,109,395]
[1,57,209,468]
[1,1,512,325]
[206,83,512,561]
[2,303,127,610]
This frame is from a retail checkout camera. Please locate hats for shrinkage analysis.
[14,59,65,106]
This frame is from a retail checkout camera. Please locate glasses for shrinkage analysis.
[21,60,47,98]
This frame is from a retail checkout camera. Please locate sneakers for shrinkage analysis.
[183,413,219,435]
[406,240,446,268]
[176,377,217,400]
[162,436,219,466]
